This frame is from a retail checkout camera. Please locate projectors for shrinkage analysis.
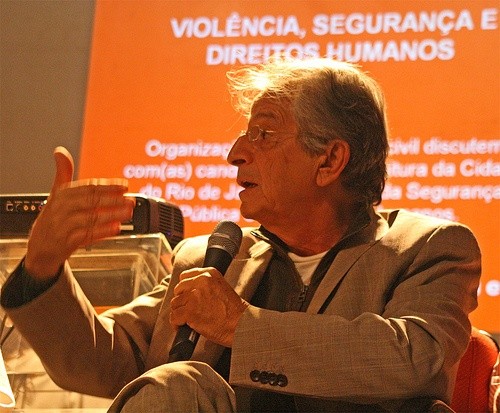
[0,194,185,251]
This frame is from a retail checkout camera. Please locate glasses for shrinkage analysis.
[237,125,324,143]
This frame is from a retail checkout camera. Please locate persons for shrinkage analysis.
[0,54,482,412]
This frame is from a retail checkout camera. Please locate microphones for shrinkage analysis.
[166,221,243,363]
